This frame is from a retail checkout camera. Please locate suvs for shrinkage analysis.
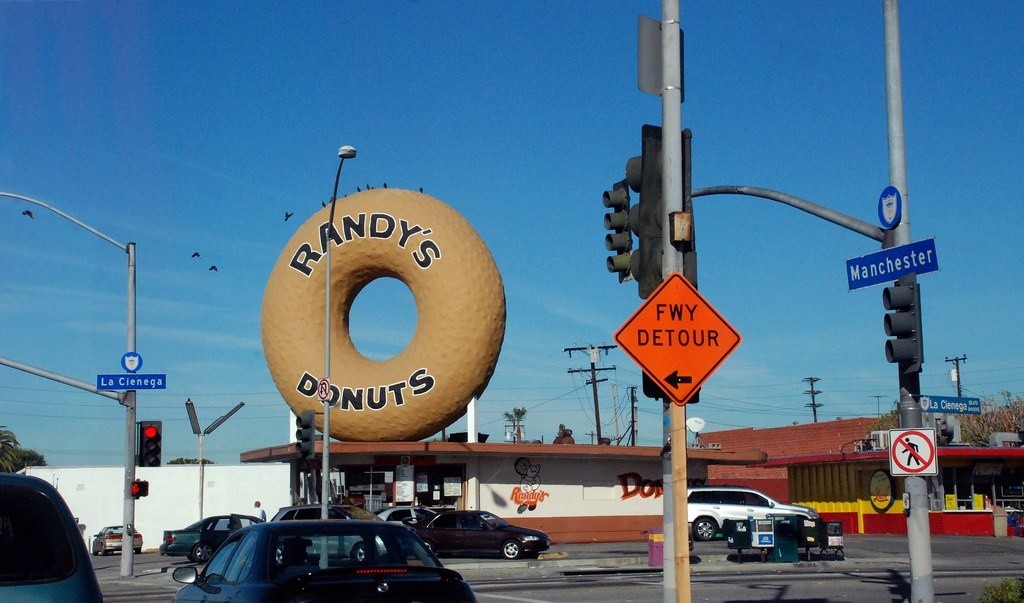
[687,488,819,540]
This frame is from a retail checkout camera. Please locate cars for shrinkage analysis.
[376,507,437,525]
[169,518,479,603]
[92,525,143,556]
[0,471,104,603]
[413,510,551,559]
[159,513,265,563]
[270,504,385,521]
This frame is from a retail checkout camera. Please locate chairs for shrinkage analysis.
[280,538,308,570]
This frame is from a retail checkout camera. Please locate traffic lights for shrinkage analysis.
[140,421,163,468]
[132,481,149,497]
[882,271,924,377]
[602,178,633,283]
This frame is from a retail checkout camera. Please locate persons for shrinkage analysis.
[1007,511,1024,538]
[249,501,266,525]
[292,498,307,506]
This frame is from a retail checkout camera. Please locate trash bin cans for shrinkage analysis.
[765,513,798,562]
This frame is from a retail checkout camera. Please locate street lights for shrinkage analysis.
[319,143,358,569]
